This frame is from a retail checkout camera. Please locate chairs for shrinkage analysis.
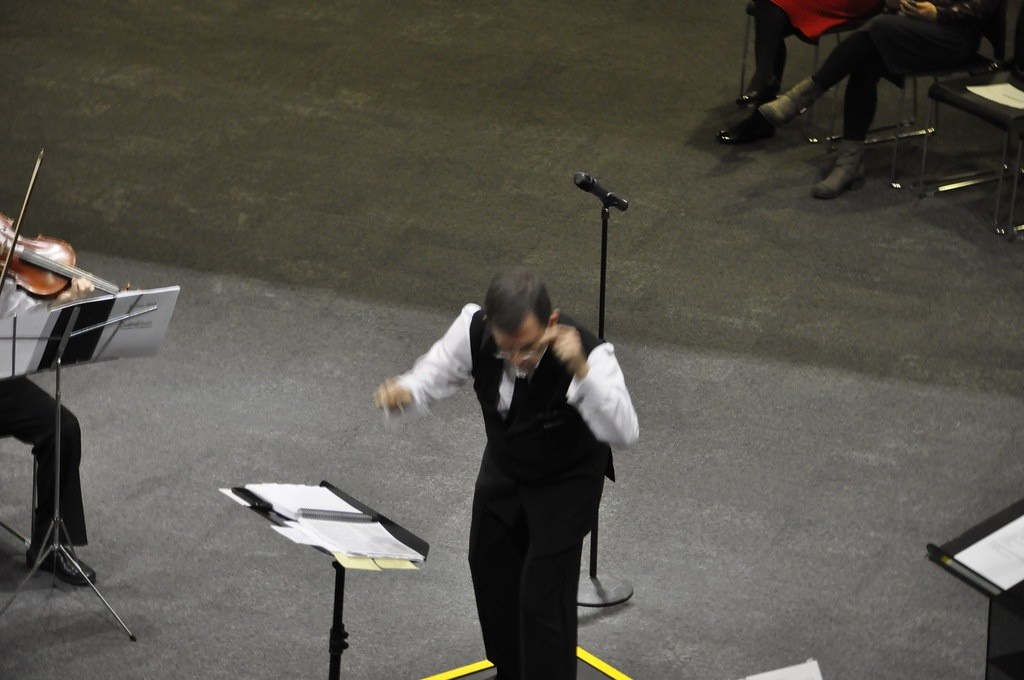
[741,0,1024,242]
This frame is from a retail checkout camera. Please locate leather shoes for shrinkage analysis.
[735,86,777,107]
[26,543,96,586]
[717,113,776,146]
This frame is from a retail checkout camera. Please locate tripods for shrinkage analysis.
[0,293,156,642]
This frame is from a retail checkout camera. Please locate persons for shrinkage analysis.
[758,0,998,198]
[0,273,95,586]
[716,0,885,145]
[372,265,640,680]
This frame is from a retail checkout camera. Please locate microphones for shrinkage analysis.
[573,172,629,212]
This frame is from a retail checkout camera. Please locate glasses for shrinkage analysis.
[491,322,543,360]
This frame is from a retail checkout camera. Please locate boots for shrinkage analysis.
[757,75,824,123]
[810,139,866,199]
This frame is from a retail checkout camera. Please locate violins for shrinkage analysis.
[0,212,129,308]
